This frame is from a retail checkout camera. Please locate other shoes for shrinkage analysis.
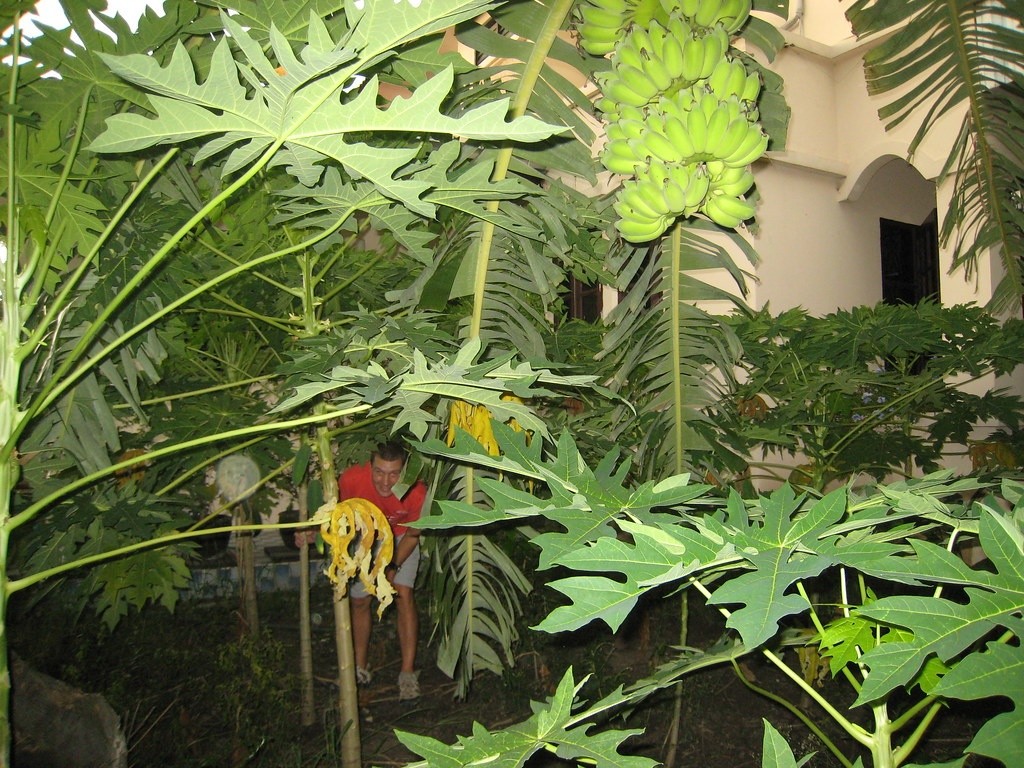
[398,671,419,700]
[355,667,371,686]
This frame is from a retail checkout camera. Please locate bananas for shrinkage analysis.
[573,0,772,242]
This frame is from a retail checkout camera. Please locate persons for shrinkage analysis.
[296,443,424,701]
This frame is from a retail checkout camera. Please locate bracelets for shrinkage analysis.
[387,563,401,571]
